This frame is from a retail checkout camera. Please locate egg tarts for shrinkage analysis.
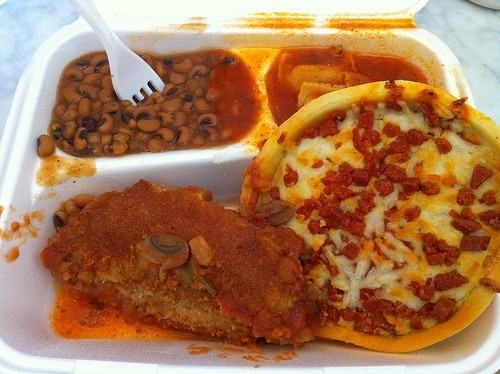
[235,76,500,352]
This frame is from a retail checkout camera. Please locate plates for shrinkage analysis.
[0,27,500,367]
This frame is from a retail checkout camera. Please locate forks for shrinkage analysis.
[67,0,166,107]
